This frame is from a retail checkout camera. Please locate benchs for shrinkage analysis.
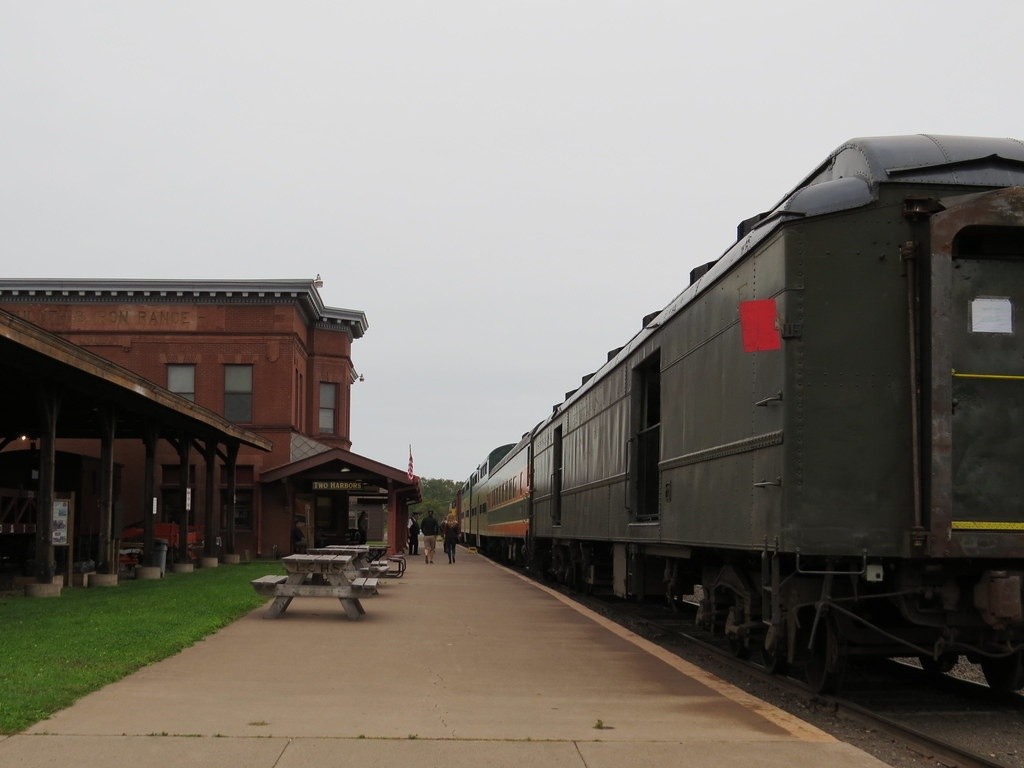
[252,545,407,620]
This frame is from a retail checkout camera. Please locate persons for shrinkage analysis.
[406,512,421,556]
[293,519,309,554]
[443,513,459,564]
[439,516,450,553]
[420,509,439,564]
[357,511,367,545]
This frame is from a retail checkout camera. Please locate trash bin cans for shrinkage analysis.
[142,538,168,579]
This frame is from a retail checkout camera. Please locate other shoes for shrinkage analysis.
[425,555,428,564]
[449,559,451,564]
[430,561,433,563]
[453,558,455,563]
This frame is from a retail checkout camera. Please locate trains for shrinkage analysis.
[446,134,1023,705]
